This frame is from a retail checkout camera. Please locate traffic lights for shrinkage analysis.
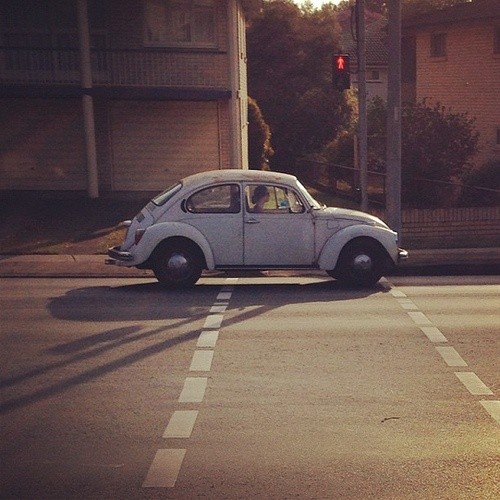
[332,52,351,90]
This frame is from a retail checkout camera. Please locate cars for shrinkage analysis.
[104,168,409,290]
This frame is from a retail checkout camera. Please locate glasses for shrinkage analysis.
[265,192,269,195]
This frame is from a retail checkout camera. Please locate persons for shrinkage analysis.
[251,185,271,212]
